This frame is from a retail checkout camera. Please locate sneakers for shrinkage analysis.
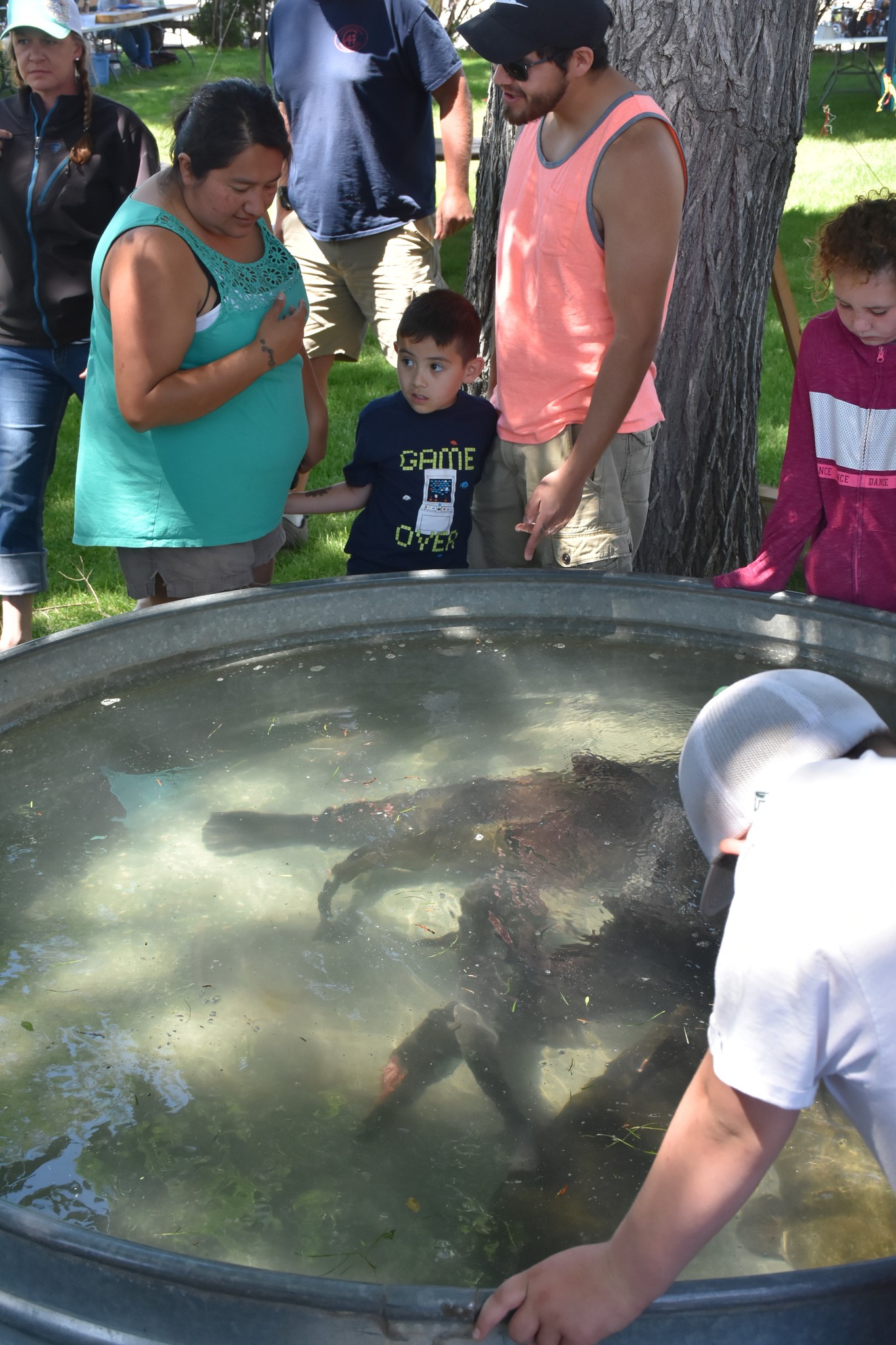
[279,515,311,548]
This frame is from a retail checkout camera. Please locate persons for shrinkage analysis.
[278,289,504,574]
[713,197,896,612]
[469,667,896,1343]
[71,79,332,608]
[96,0,157,75]
[0,0,163,653]
[459,0,689,576]
[265,0,475,552]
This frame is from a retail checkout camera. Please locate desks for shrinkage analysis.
[813,36,888,108]
[80,4,200,83]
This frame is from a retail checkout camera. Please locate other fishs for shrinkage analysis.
[200,744,734,1280]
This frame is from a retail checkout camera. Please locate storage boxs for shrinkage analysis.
[87,54,110,86]
[80,14,96,28]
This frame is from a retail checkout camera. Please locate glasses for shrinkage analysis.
[494,51,559,81]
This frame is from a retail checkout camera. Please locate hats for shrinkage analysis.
[0,0,84,40]
[454,0,613,65]
[678,669,890,919]
